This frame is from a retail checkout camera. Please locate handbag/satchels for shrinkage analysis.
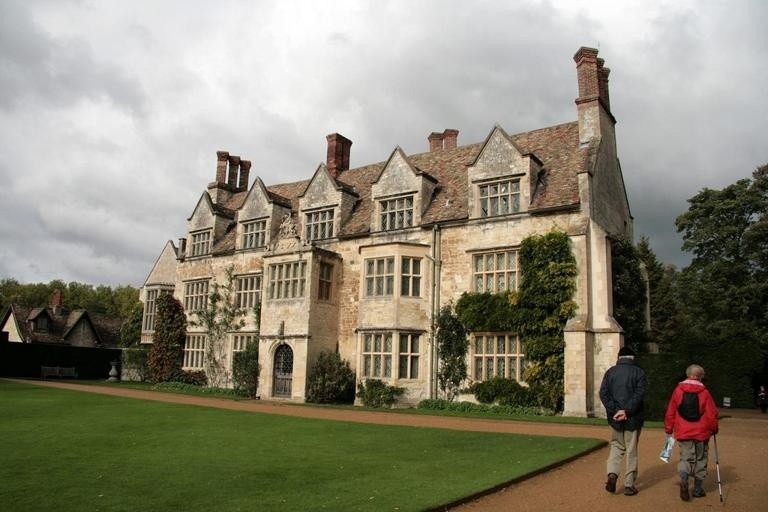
[658,434,675,463]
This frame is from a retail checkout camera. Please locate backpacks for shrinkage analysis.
[677,392,702,422]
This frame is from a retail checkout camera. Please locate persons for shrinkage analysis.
[664,363,722,501]
[599,345,648,495]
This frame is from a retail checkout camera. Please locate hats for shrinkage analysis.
[618,346,637,356]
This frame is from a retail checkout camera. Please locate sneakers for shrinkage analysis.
[623,486,638,495]
[605,473,617,492]
[691,486,706,497]
[679,481,689,501]
